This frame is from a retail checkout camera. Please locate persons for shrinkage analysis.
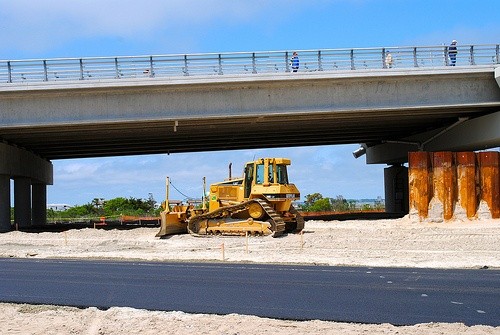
[448,40,458,67]
[289,52,300,73]
[384,50,393,68]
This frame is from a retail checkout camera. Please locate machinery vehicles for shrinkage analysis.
[155,157,305,238]
